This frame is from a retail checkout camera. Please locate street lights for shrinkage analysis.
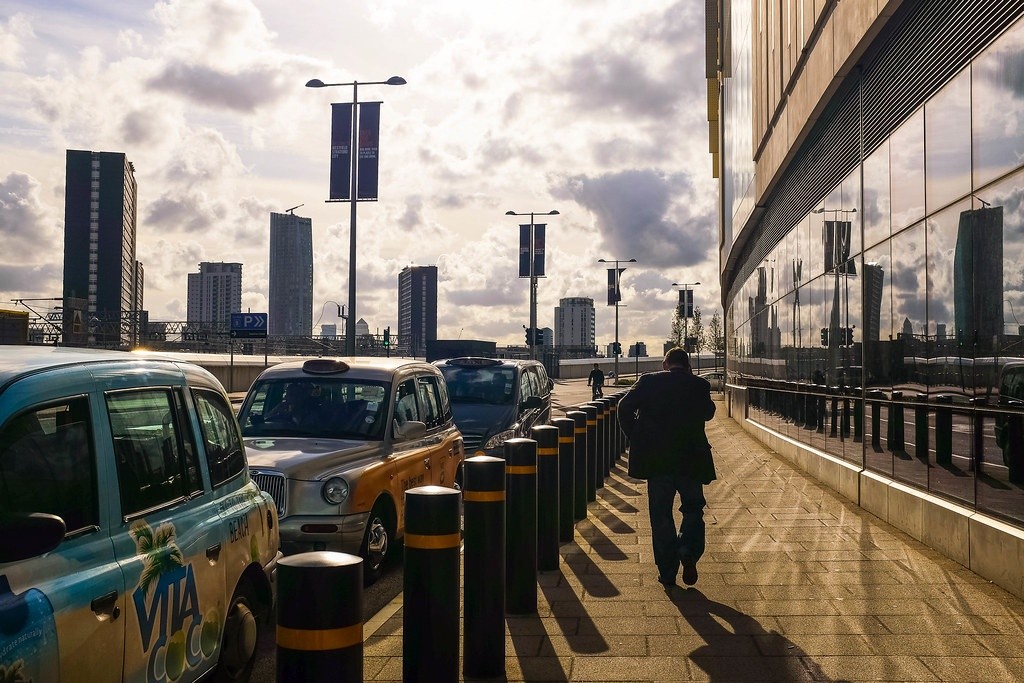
[598,258,637,386]
[306,75,407,357]
[505,210,560,359]
[672,282,701,351]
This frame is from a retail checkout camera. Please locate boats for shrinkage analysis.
[0,344,286,683]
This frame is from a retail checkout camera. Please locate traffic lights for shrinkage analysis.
[613,342,618,354]
[525,328,533,345]
[535,328,544,345]
[618,343,622,354]
[636,343,641,355]
[385,329,390,345]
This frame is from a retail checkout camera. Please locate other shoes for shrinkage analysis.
[682,553,698,586]
[657,575,676,587]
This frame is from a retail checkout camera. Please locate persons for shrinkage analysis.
[263,384,324,432]
[588,363,604,400]
[812,363,825,386]
[617,348,717,585]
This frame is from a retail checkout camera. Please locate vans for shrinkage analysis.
[432,357,553,463]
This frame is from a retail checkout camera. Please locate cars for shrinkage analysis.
[237,356,464,580]
[698,372,724,391]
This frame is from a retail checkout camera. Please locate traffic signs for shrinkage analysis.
[229,337,268,344]
[230,330,268,338]
